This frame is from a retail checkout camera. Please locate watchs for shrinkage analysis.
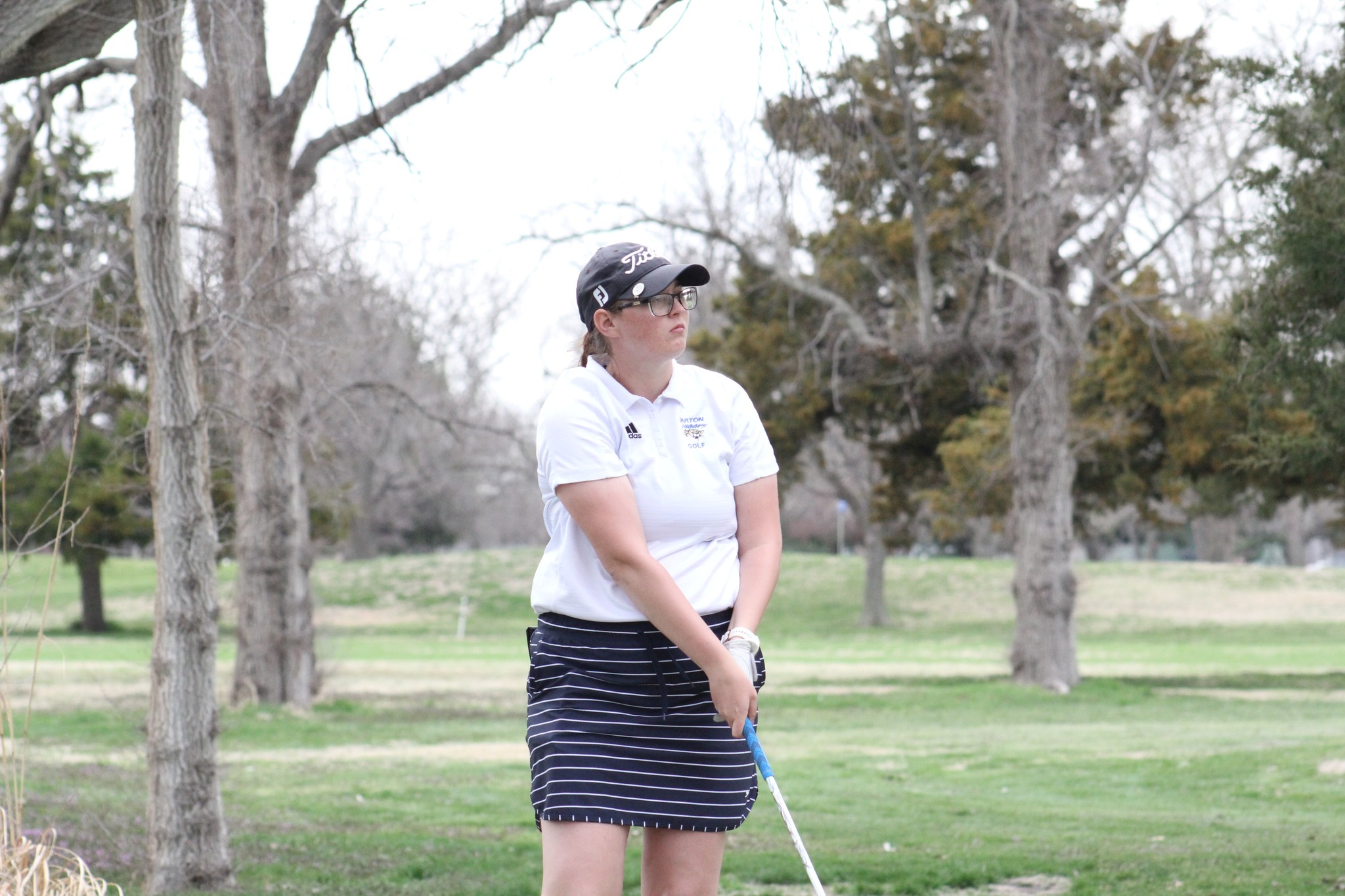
[721,627,760,657]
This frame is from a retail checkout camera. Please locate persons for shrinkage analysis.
[525,243,782,896]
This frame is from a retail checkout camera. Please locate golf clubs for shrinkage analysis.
[743,717,826,896]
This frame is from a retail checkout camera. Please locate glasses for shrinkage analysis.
[618,287,702,316]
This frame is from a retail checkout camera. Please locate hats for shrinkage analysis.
[576,242,710,334]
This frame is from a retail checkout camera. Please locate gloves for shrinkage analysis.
[713,639,758,722]
